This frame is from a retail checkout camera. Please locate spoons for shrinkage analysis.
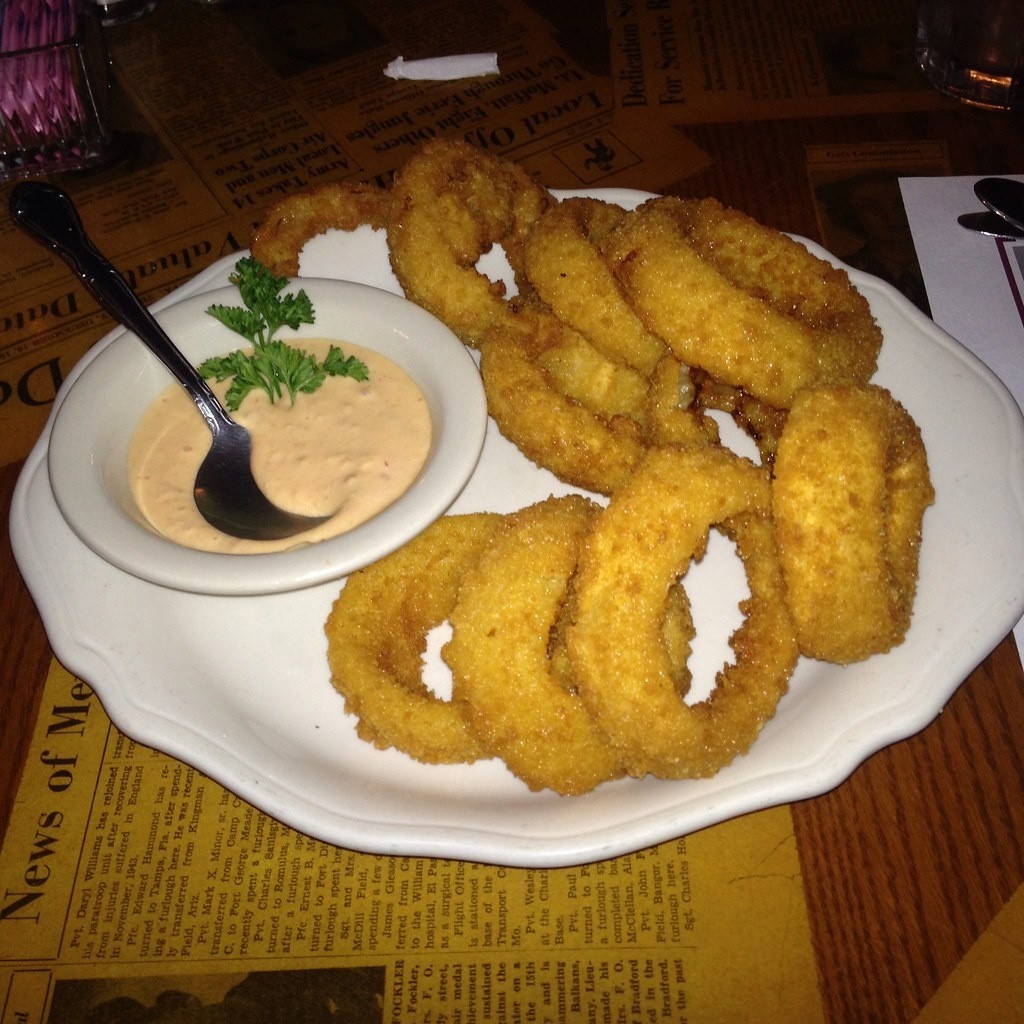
[8,175,334,540]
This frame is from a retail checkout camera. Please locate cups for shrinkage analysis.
[917,1,1024,115]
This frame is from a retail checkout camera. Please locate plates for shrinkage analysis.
[48,275,489,598]
[8,183,1022,873]
